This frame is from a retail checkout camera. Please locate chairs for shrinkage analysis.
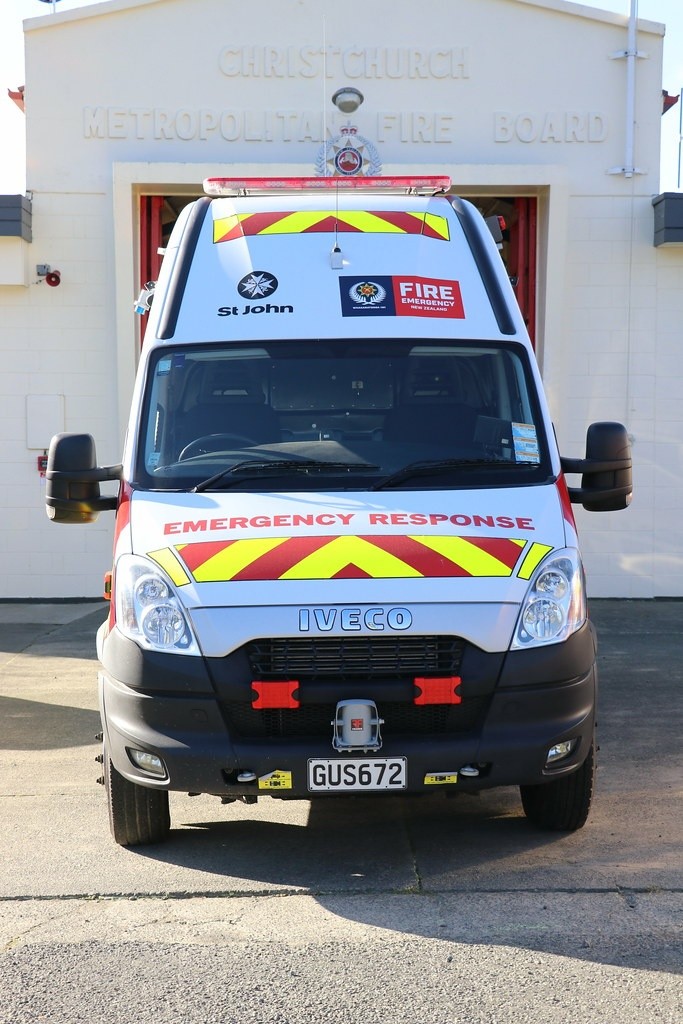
[381,356,486,447]
[177,363,276,457]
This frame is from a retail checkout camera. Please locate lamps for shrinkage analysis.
[331,86,365,114]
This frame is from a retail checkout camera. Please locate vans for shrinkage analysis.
[45,173,635,850]
